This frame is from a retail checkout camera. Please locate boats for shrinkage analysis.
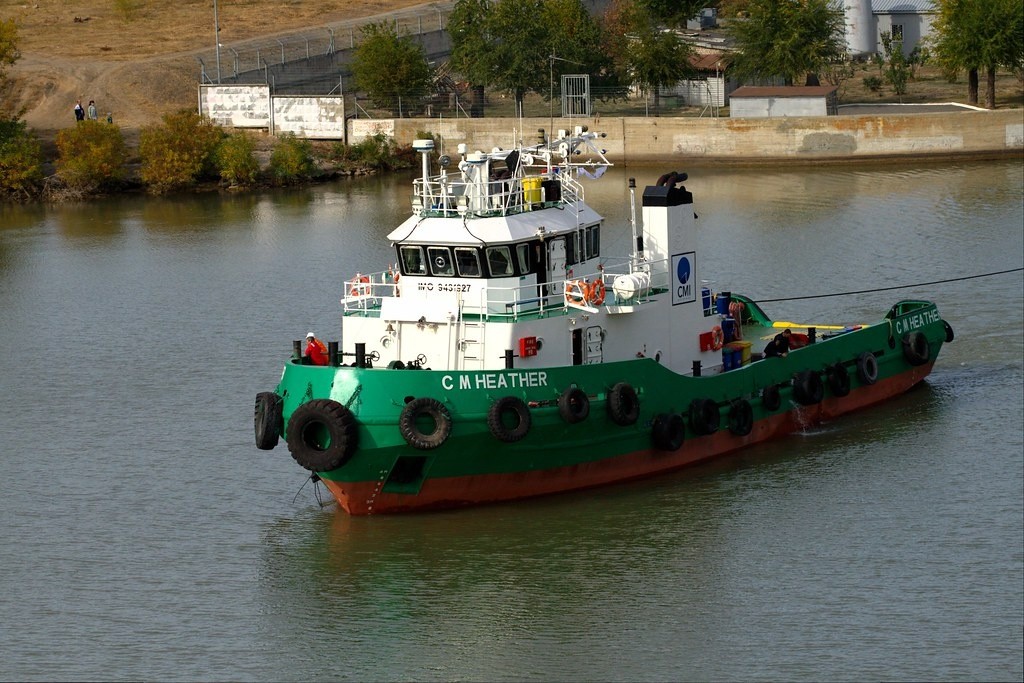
[254,55,954,515]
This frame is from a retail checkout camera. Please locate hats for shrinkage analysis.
[306,332,314,338]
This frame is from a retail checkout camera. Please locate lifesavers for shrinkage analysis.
[793,370,824,405]
[655,414,685,452]
[608,383,641,426]
[907,330,931,365]
[487,396,533,443]
[591,281,606,306]
[399,397,452,448]
[566,280,588,305]
[765,383,782,411]
[828,363,851,398]
[711,325,725,351]
[253,393,282,449]
[939,319,955,344]
[728,399,754,437]
[859,352,878,383]
[352,276,369,298]
[285,399,359,473]
[690,398,720,436]
[558,389,589,425]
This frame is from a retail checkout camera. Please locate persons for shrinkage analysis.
[88,100,98,120]
[773,328,791,354]
[74,99,86,122]
[304,332,329,366]
[106,112,113,124]
[763,335,787,359]
